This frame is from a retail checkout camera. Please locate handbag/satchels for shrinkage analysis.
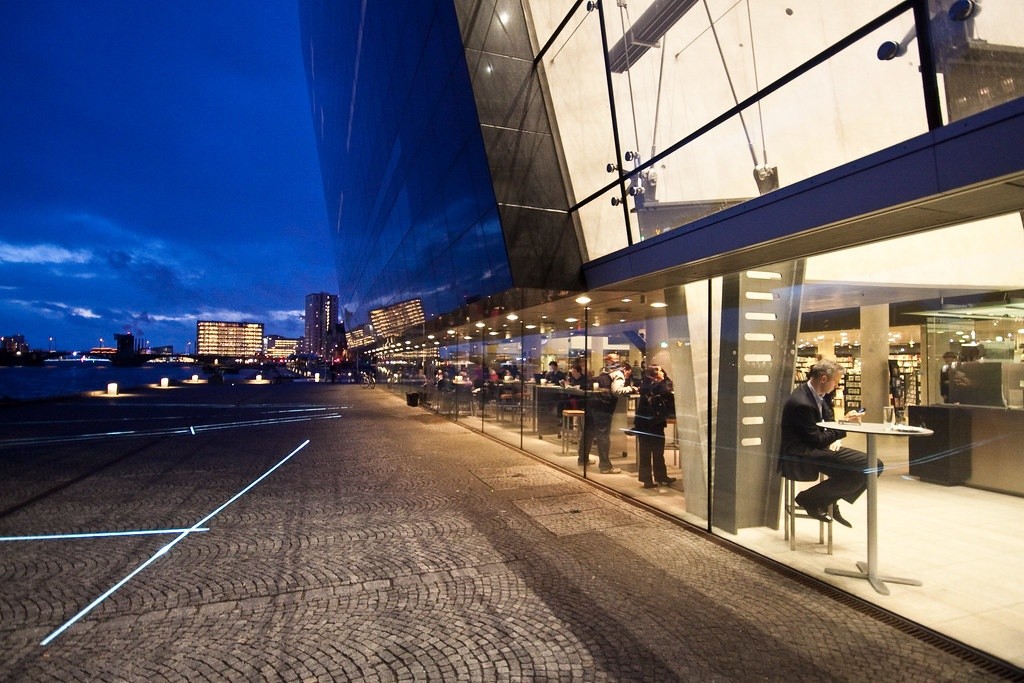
[647,391,673,415]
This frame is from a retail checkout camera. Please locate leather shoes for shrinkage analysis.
[795,491,833,522]
[824,503,852,528]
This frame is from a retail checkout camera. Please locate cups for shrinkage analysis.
[593,382,599,392]
[540,379,546,387]
[882,407,895,426]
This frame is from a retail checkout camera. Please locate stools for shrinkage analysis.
[422,376,680,481]
[780,461,835,556]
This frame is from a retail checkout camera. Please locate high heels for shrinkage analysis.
[643,480,658,489]
[656,476,676,485]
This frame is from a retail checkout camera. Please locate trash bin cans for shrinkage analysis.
[406,392,419,407]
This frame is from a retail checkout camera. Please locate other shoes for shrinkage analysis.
[602,466,622,473]
[558,418,572,426]
[577,459,597,465]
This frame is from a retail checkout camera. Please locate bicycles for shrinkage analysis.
[359,370,376,389]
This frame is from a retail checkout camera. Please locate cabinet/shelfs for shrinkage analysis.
[799,354,922,417]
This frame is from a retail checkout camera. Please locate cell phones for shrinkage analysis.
[858,408,866,413]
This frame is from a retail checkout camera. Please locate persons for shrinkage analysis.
[940,352,959,403]
[413,353,676,489]
[776,358,884,528]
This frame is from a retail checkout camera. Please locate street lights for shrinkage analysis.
[99,337,103,354]
[185,341,191,355]
[145,340,149,354]
[48,337,53,352]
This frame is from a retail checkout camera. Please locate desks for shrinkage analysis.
[815,422,936,595]
[535,383,629,459]
